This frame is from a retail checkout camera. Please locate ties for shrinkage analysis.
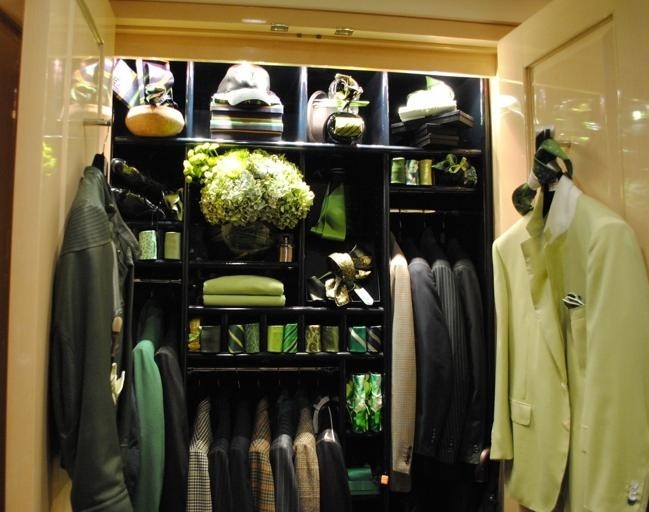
[189,319,382,355]
[512,138,573,215]
[391,157,433,186]
[139,229,181,261]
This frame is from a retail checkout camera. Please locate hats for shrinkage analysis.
[225,63,271,107]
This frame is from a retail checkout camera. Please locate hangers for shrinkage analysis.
[189,362,341,462]
[390,205,478,279]
[492,135,633,253]
[74,115,117,230]
[132,275,176,359]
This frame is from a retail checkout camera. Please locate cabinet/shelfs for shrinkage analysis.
[106,49,498,512]
